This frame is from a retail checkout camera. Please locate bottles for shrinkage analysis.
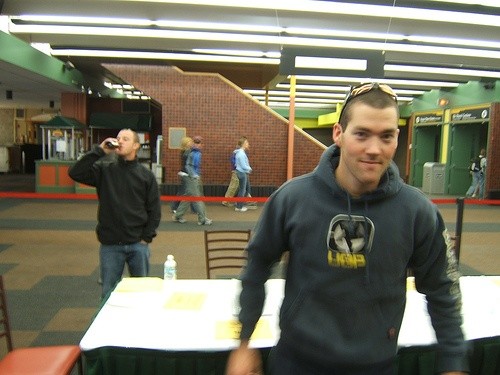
[106,141,121,149]
[164,255,176,292]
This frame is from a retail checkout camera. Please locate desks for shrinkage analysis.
[78,276,500,375]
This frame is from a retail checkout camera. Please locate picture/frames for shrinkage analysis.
[168,127,186,150]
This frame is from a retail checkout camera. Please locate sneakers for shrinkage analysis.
[198,217,214,225]
[172,216,187,224]
[222,201,237,207]
[247,205,257,209]
[170,210,176,214]
[235,207,247,212]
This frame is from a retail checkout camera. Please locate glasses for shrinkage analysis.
[338,82,397,124]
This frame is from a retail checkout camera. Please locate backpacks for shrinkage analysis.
[470,155,485,172]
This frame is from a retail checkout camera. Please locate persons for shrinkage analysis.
[465,148,486,200]
[97,135,256,287]
[68,128,161,302]
[224,83,474,375]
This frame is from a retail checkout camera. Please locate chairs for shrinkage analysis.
[0,275,83,375]
[204,230,252,279]
